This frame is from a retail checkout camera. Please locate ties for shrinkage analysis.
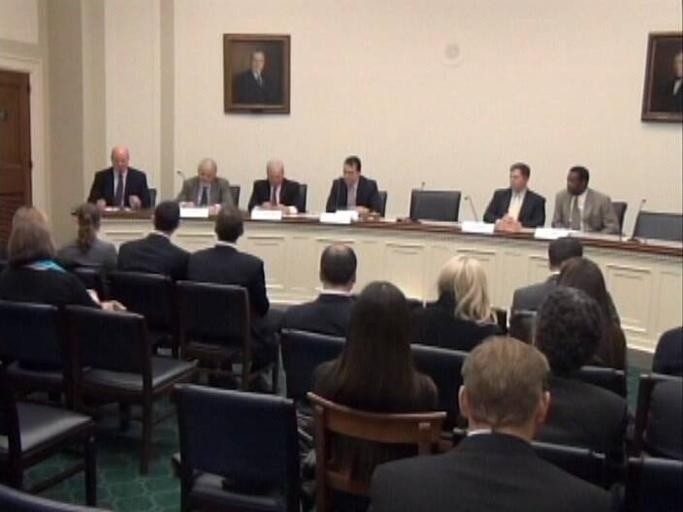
[272,186,278,207]
[570,195,581,231]
[199,186,208,207]
[115,173,123,206]
[510,193,521,220]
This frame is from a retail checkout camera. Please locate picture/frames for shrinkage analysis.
[641,32,683,124]
[224,33,290,112]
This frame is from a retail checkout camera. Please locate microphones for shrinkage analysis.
[177,170,189,207]
[410,181,425,221]
[464,194,478,220]
[626,199,647,243]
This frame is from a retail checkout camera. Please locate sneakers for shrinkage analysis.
[222,374,267,393]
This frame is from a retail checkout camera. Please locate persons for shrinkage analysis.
[662,47,683,112]
[278,245,358,345]
[84,144,150,211]
[512,236,682,456]
[4,203,127,313]
[482,162,546,227]
[549,166,621,234]
[314,281,438,412]
[372,335,612,512]
[116,203,282,396]
[325,156,381,217]
[234,50,277,102]
[246,160,303,214]
[175,158,233,216]
[413,252,505,352]
